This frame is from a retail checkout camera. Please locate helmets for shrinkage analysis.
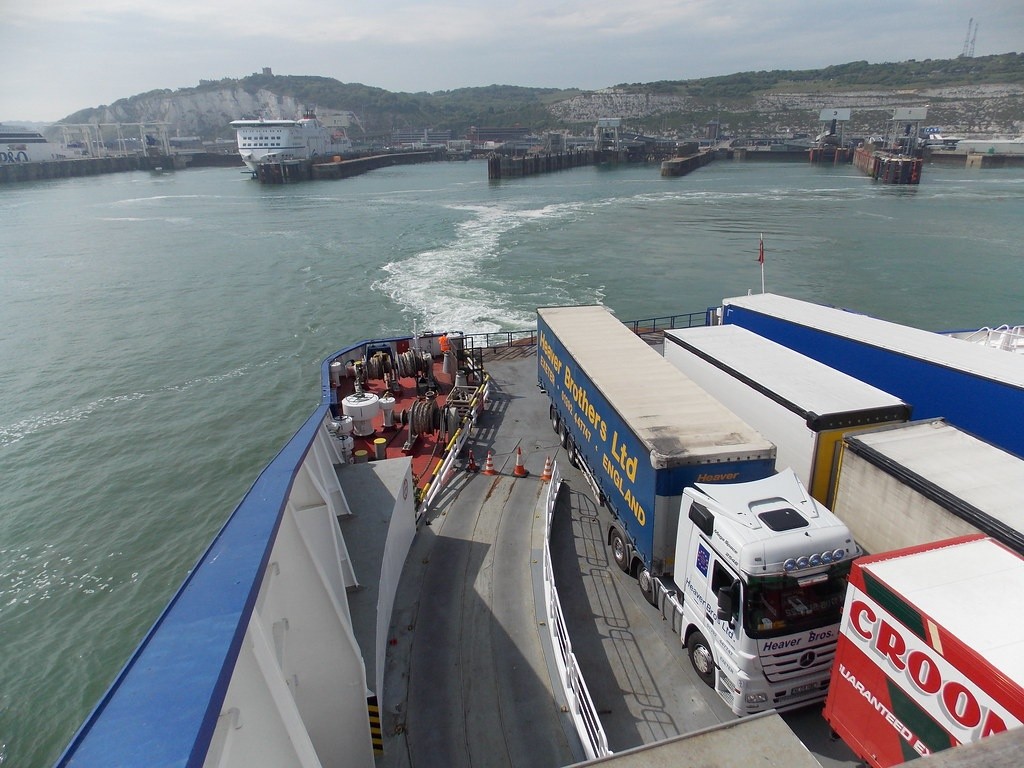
[443,330,448,334]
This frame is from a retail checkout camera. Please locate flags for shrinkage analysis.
[759,240,763,265]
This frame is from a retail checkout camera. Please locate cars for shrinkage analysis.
[919,127,939,138]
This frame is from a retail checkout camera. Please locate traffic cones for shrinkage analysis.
[511,446,529,478]
[540,456,552,481]
[466,448,479,472]
[484,451,496,475]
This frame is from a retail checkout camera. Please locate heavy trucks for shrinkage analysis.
[536,303,864,718]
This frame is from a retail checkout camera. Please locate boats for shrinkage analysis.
[228,109,353,173]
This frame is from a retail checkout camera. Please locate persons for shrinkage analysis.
[439,333,451,362]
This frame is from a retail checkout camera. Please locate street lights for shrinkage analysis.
[918,105,930,137]
